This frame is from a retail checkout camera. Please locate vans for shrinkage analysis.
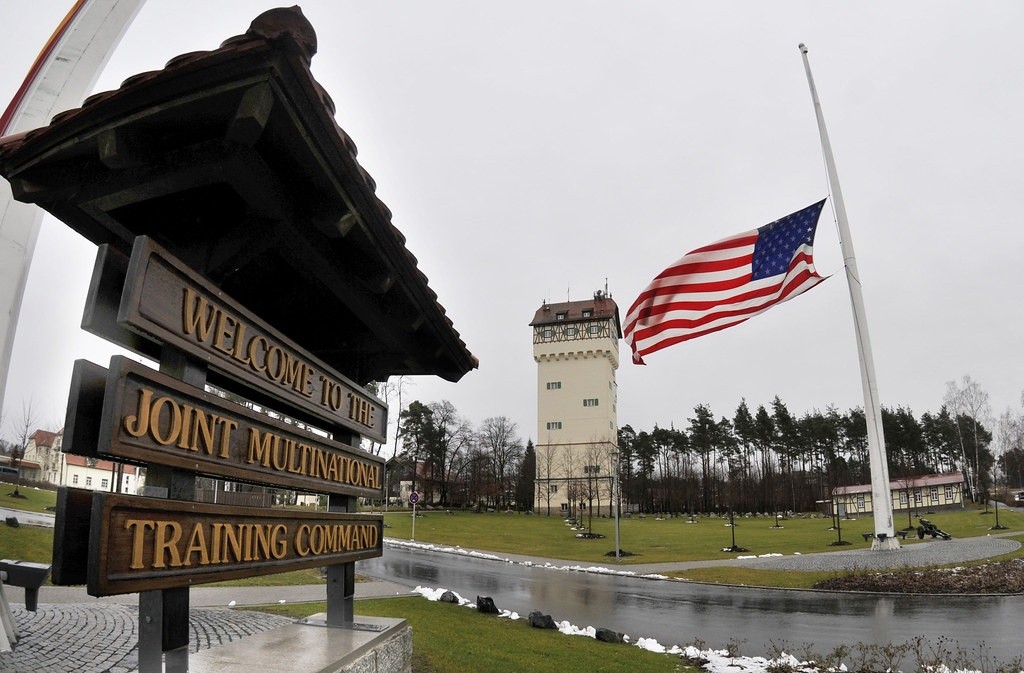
[0,466,18,481]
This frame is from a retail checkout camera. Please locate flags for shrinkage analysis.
[623,196,833,365]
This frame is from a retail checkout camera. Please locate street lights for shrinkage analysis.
[609,448,622,557]
